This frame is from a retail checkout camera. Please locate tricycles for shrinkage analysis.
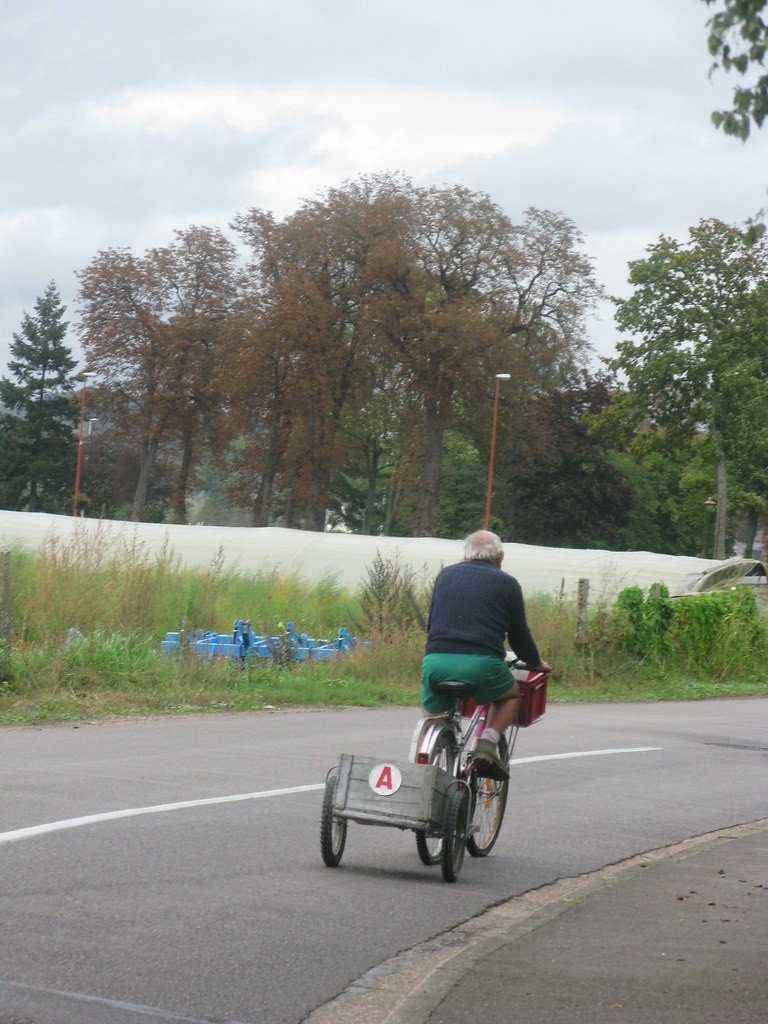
[318,654,555,883]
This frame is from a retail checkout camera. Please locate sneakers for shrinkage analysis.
[475,737,511,783]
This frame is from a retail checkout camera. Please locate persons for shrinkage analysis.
[422,522,551,778]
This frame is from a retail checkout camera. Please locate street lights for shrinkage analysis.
[74,373,96,517]
[484,374,512,531]
